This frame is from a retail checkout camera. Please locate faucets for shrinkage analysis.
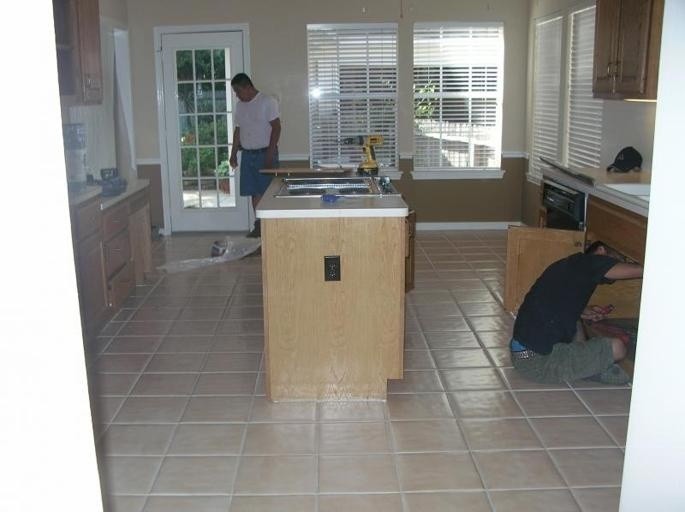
[379,175,391,192]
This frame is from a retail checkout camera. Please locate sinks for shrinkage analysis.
[278,185,373,198]
[282,175,372,185]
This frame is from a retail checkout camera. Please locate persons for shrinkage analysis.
[508,242,644,385]
[228,72,281,238]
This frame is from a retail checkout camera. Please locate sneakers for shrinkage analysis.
[245,219,261,239]
[584,361,631,385]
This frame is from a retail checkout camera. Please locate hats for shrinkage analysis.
[606,145,642,173]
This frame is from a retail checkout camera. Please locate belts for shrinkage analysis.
[508,347,539,362]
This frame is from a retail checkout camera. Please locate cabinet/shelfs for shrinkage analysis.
[590,0,664,103]
[71,197,107,334]
[52,1,104,106]
[101,190,152,305]
[503,196,648,384]
